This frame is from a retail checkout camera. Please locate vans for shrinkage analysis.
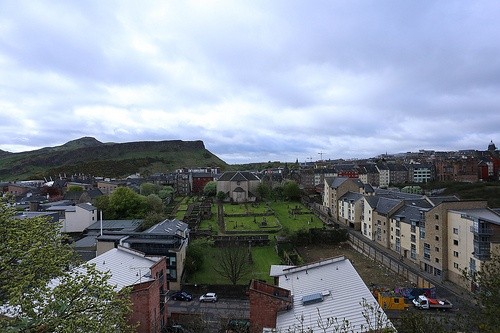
[170,291,192,302]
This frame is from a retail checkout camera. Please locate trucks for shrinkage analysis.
[413,295,453,310]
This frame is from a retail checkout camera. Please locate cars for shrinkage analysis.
[199,292,217,303]
